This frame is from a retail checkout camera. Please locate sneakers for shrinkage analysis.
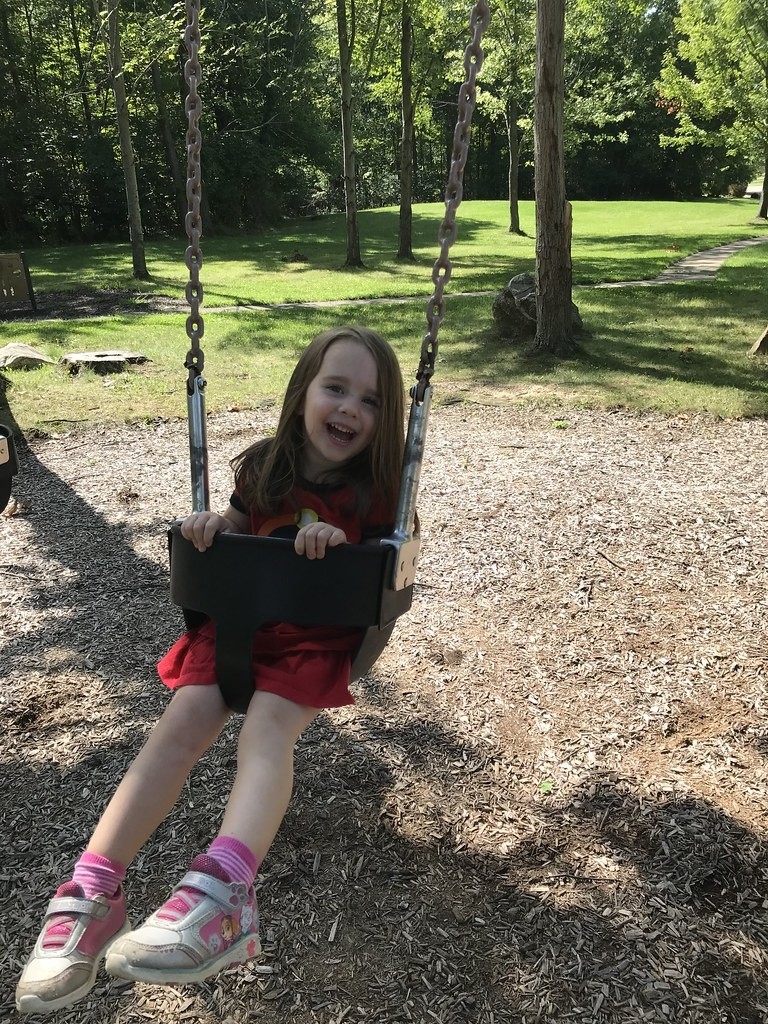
[15,880,131,1013]
[103,854,261,986]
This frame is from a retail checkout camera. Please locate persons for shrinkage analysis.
[15,326,405,1013]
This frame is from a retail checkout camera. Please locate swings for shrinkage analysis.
[166,0,494,717]
